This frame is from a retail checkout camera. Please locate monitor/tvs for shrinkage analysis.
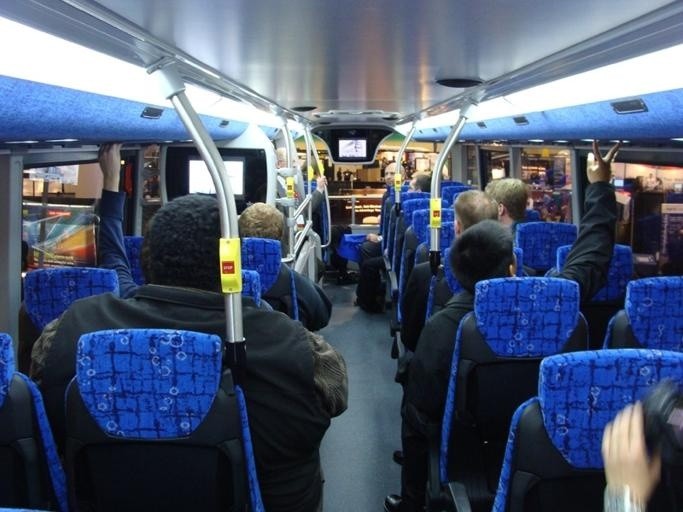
[188,155,245,201]
[338,139,367,158]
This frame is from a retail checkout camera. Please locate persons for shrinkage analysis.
[344,168,356,189]
[94,142,153,300]
[29,192,349,511]
[236,201,334,334]
[336,167,342,187]
[276,146,329,283]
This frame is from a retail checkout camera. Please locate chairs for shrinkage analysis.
[301,180,330,264]
[377,179,681,510]
[1,233,282,512]
